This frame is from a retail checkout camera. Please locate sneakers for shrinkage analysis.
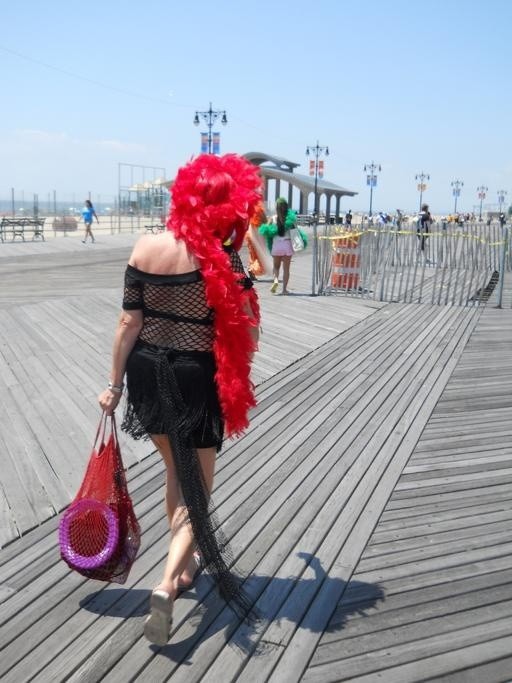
[271,283,279,293]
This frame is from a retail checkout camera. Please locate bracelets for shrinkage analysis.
[106,380,125,392]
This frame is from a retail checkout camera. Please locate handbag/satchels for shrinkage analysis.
[290,226,308,252]
[57,405,141,585]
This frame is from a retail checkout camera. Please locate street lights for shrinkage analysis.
[305,139,331,224]
[363,160,382,217]
[193,100,229,156]
[450,178,508,218]
[414,171,430,213]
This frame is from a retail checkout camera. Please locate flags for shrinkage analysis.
[366,175,370,186]
[319,160,324,179]
[200,132,208,154]
[212,132,221,155]
[373,175,377,187]
[308,159,315,176]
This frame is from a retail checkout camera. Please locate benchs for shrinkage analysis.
[1,216,47,243]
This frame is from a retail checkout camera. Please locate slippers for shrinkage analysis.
[142,590,174,647]
[175,552,204,591]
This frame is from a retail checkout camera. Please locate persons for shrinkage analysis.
[77,199,100,241]
[95,151,265,648]
[244,195,505,296]
